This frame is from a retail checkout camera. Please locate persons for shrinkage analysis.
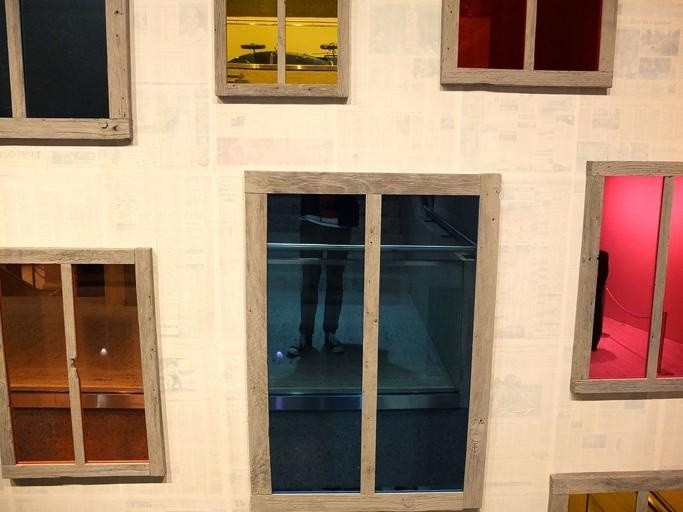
[288,195,360,357]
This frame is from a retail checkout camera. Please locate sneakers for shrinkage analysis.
[324,332,343,352]
[288,335,312,355]
